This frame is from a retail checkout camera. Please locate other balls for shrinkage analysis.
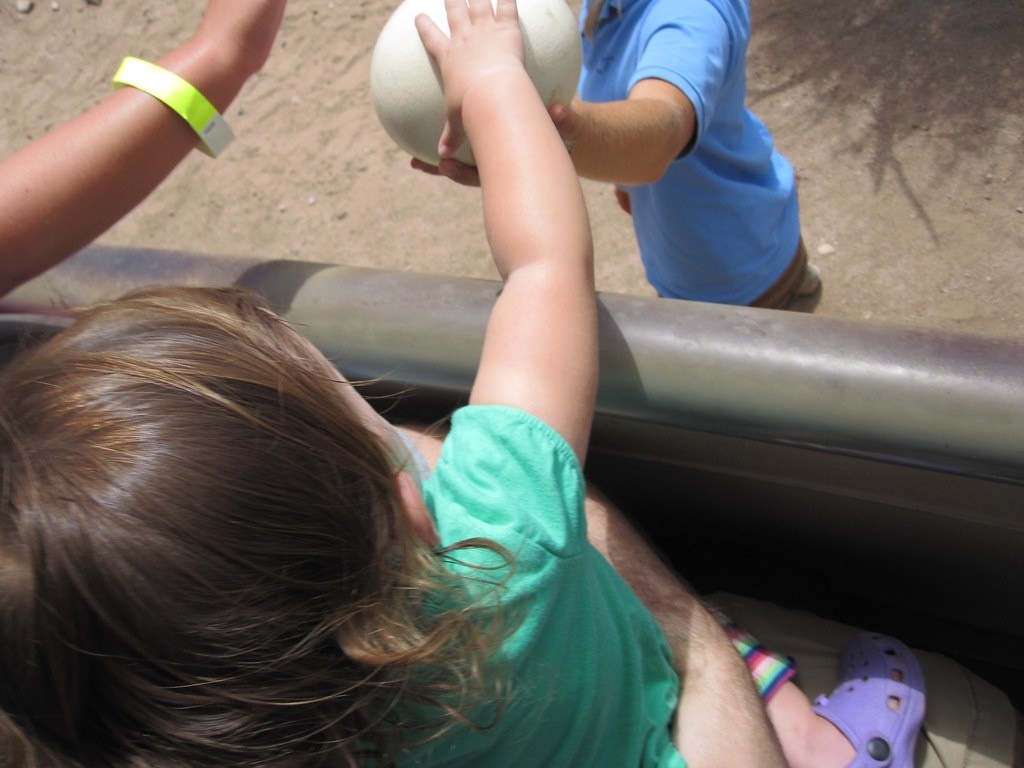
[366,0,582,168]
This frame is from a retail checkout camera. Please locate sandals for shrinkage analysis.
[811,632,926,768]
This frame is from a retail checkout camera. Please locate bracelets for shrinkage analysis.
[113,57,236,159]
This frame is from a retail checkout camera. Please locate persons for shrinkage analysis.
[0,1,284,299]
[410,1,821,312]
[0,0,924,768]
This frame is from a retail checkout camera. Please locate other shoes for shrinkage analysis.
[797,264,821,295]
